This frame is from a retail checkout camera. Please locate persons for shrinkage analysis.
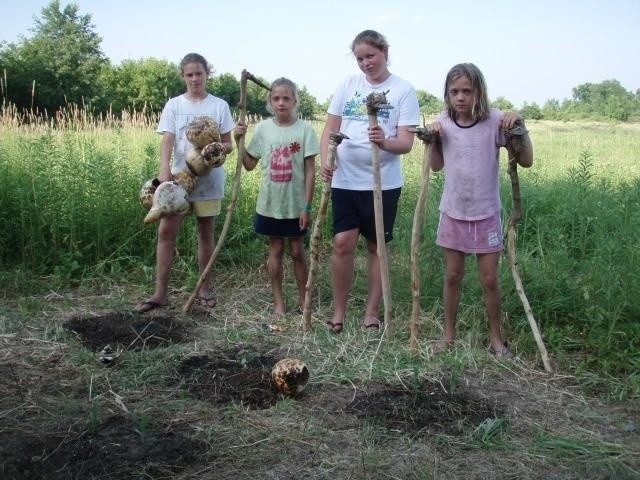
[235,77,320,318]
[427,63,533,362]
[135,53,234,312]
[320,30,420,335]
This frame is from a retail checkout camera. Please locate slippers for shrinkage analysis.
[132,300,168,315]
[431,339,452,354]
[488,342,515,363]
[198,294,217,308]
[325,320,347,335]
[362,319,380,330]
